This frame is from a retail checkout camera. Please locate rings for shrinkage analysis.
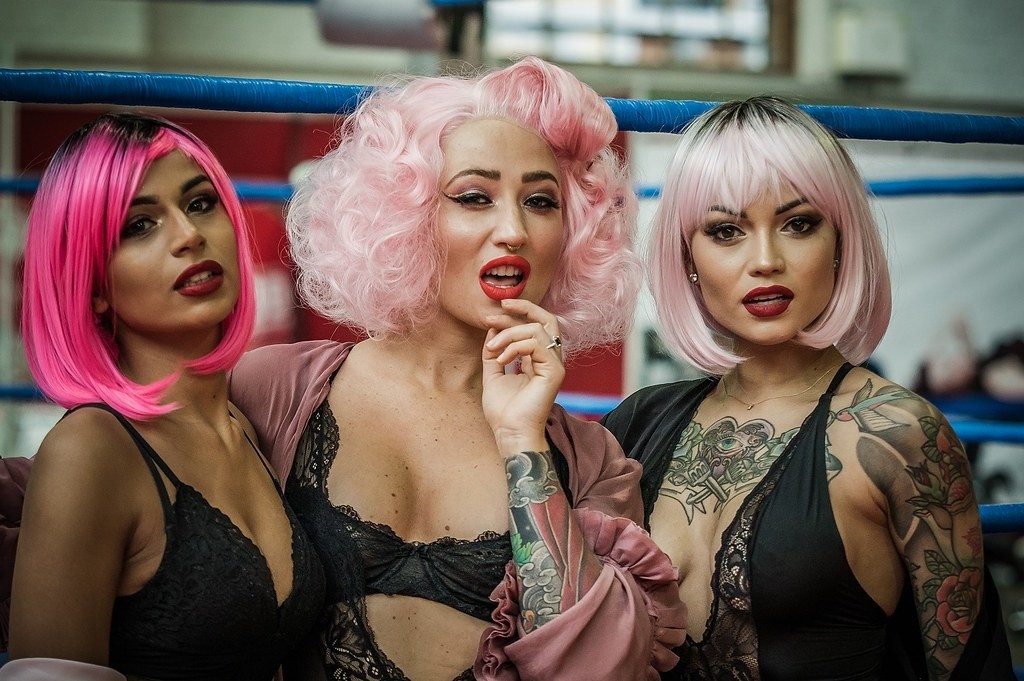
[545,336,562,350]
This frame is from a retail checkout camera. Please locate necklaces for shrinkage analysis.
[718,358,846,411]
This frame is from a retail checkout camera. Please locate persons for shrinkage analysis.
[0,109,327,681]
[592,92,1017,681]
[0,50,697,681]
